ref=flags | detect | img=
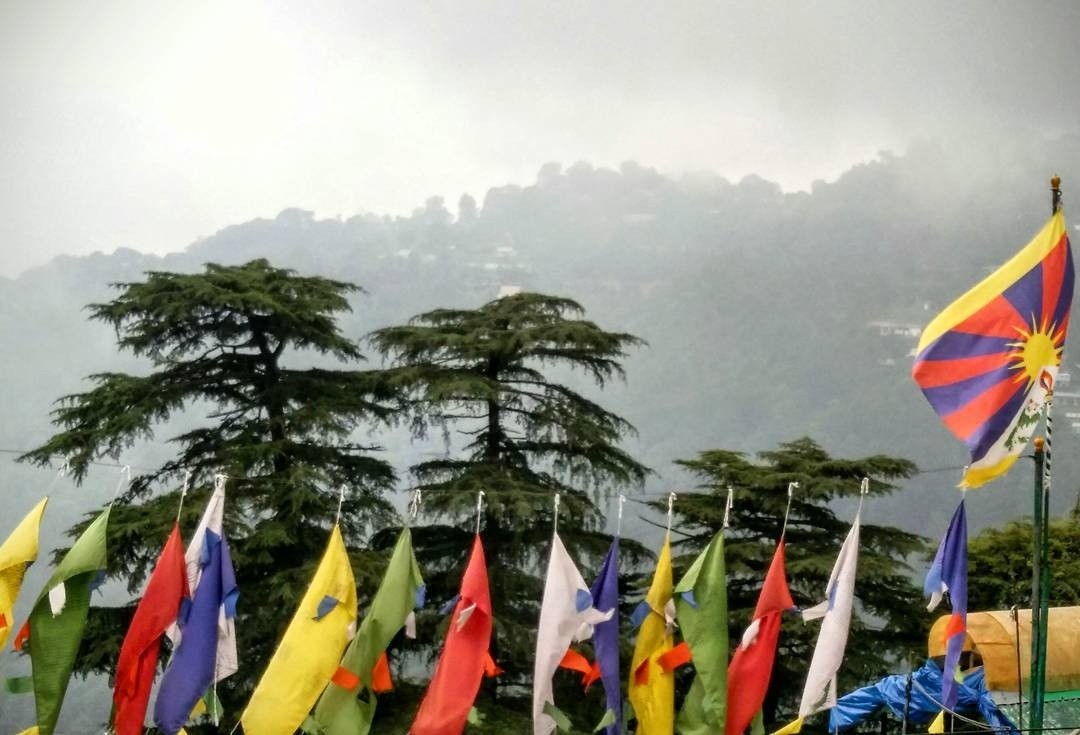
[674,524,727,735]
[770,511,860,735]
[913,207,1075,488]
[533,532,598,735]
[302,522,426,735]
[927,498,969,711]
[153,481,238,735]
[587,535,628,735]
[241,523,358,735]
[724,541,793,735]
[627,532,676,735]
[113,525,189,735]
[408,535,492,735]
[27,504,110,735]
[0,497,48,655]
